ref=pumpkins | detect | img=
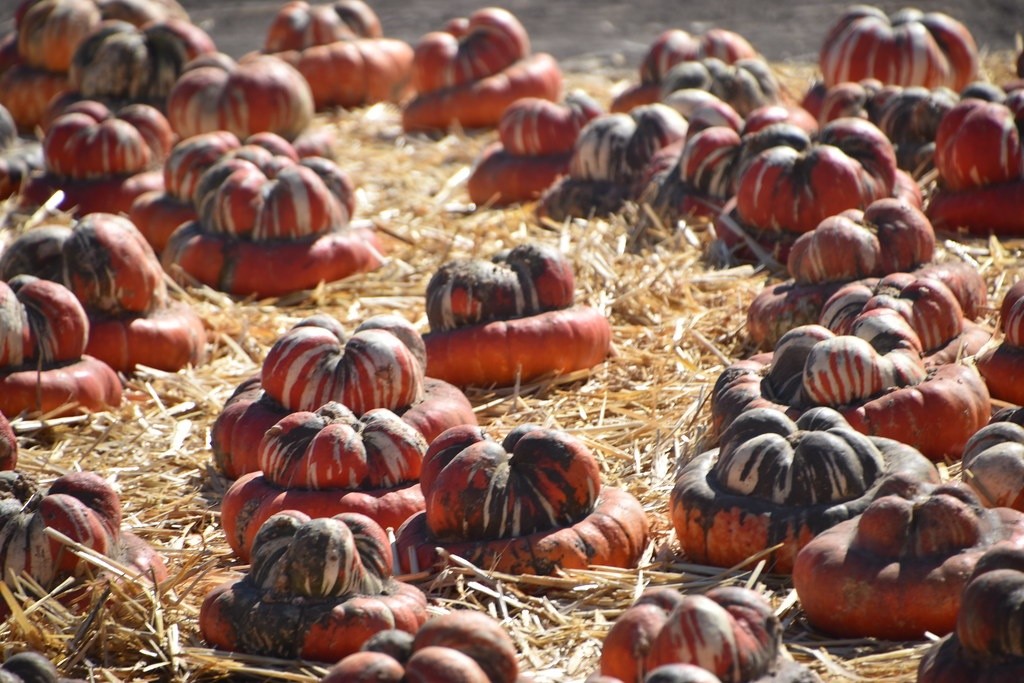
[0,0,1024,683]
